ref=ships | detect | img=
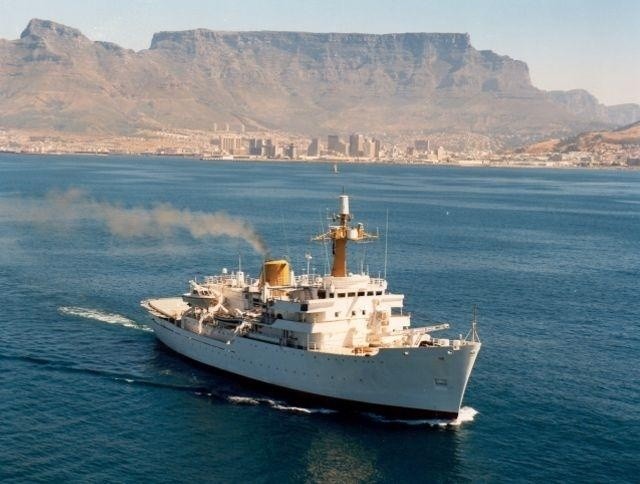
[136,184,482,426]
